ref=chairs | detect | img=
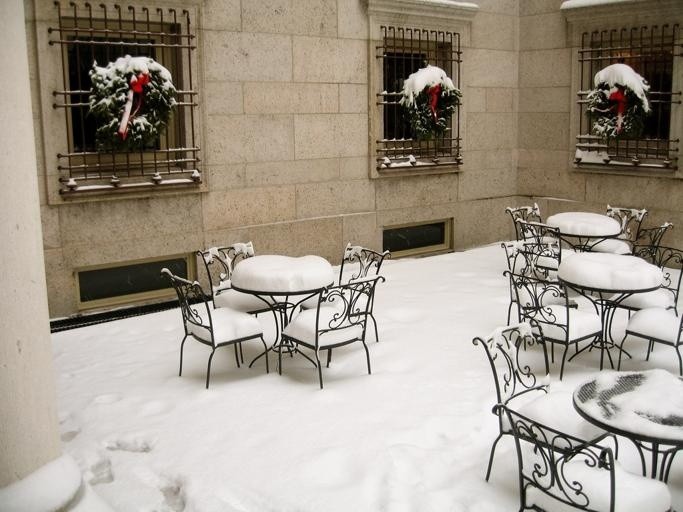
[160,239,392,390]
